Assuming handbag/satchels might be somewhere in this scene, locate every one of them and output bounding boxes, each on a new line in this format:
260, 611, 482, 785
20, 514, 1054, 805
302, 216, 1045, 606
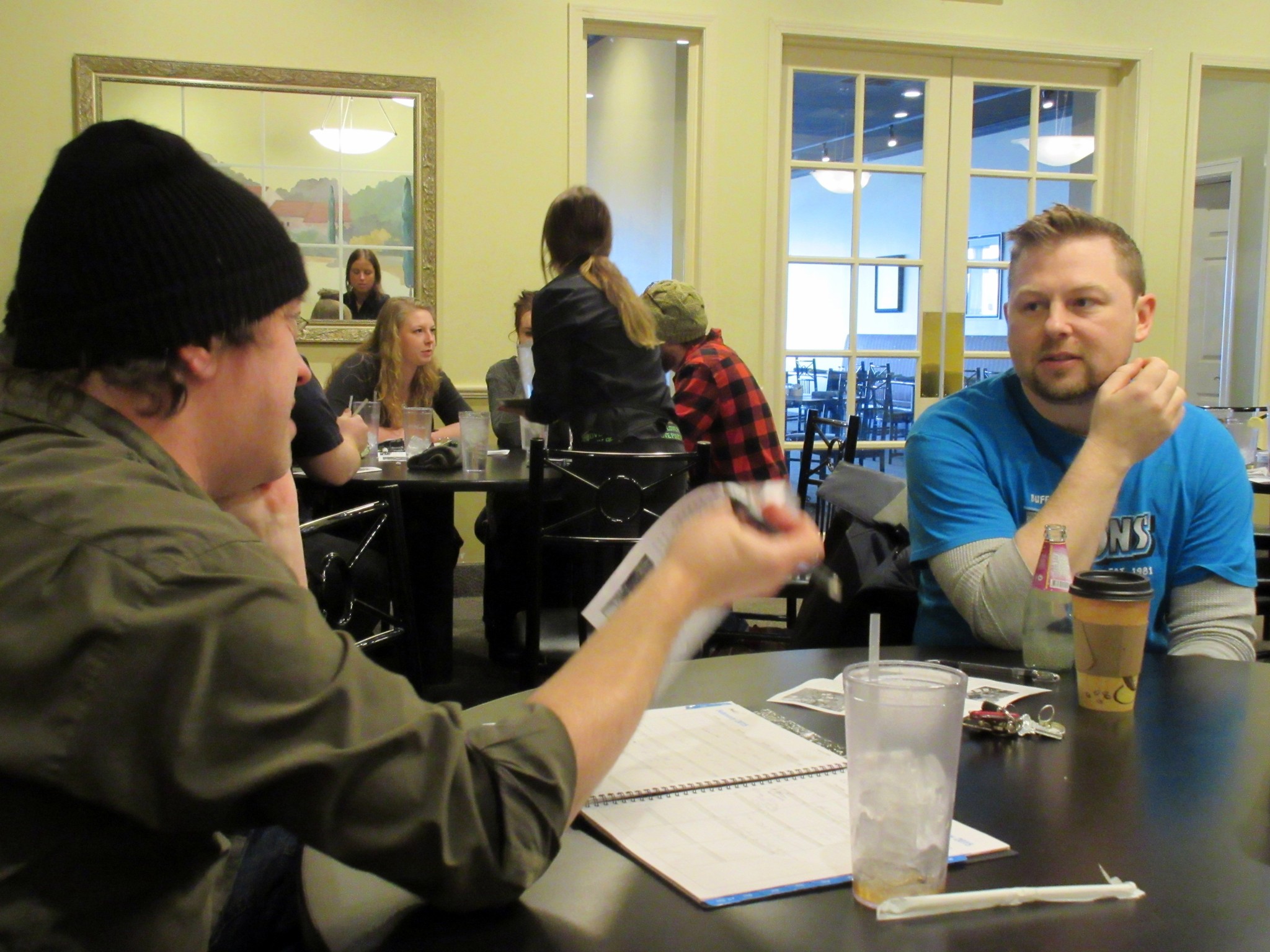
782, 508, 921, 649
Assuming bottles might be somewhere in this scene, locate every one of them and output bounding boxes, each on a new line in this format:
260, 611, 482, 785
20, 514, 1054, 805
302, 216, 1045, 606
1020, 524, 1075, 671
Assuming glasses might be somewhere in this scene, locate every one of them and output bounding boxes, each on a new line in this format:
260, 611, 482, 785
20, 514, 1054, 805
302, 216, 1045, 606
643, 281, 666, 317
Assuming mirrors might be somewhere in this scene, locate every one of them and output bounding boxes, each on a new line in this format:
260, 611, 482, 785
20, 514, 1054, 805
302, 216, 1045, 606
73, 54, 436, 343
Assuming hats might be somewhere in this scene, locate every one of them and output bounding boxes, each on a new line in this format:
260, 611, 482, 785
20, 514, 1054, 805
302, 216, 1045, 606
2, 118, 310, 374
640, 279, 708, 344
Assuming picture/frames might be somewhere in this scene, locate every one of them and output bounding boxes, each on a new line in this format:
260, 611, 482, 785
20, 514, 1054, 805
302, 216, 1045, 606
875, 254, 903, 313
966, 233, 1003, 318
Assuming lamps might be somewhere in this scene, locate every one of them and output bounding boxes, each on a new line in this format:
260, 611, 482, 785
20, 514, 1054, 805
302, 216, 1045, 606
1011, 90, 1095, 166
809, 124, 871, 193
308, 96, 397, 154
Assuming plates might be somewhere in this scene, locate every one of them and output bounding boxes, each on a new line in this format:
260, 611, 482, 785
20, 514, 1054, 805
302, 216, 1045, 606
1249, 478, 1270, 484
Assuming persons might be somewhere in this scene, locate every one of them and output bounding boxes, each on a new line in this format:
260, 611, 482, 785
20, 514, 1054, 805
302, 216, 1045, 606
906, 204, 1259, 660
0, 119, 828, 952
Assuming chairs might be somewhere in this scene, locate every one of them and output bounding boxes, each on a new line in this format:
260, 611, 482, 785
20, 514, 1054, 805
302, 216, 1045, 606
521, 356, 999, 680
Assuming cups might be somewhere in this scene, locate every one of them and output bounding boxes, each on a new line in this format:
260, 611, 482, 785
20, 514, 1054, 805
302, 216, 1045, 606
459, 411, 490, 473
843, 660, 969, 910
1226, 419, 1259, 469
351, 401, 381, 459
400, 407, 434, 461
1069, 569, 1156, 714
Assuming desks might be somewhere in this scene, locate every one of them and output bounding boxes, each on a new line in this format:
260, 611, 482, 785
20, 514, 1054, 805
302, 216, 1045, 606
289, 436, 556, 487
786, 394, 865, 432
300, 646, 1270, 952
